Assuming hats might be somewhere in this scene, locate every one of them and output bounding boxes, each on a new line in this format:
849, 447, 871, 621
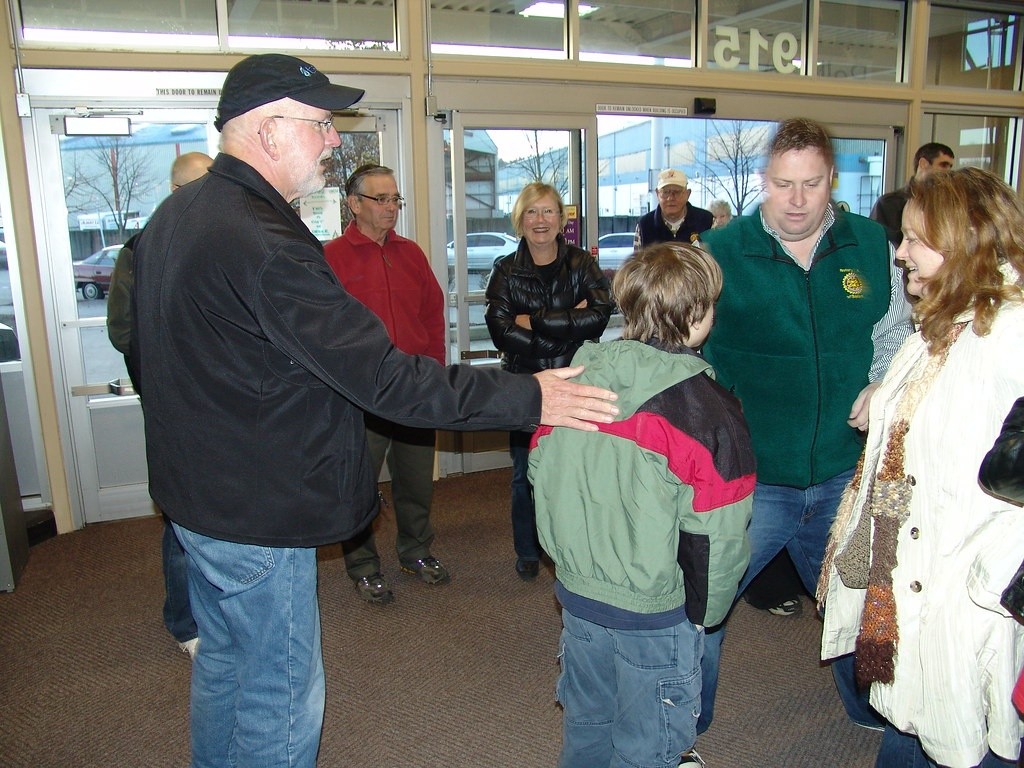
213, 53, 366, 133
656, 169, 688, 191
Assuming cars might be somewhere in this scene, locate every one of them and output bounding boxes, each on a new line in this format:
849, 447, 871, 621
582, 233, 638, 274
0, 241, 8, 269
0, 323, 21, 362
447, 230, 524, 276
73, 243, 126, 300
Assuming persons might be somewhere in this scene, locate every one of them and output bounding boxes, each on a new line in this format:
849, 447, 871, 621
633, 169, 713, 253
677, 119, 914, 768
709, 200, 733, 230
744, 141, 1024, 767
129, 53, 620, 768
526, 240, 755, 768
318, 165, 450, 609
107, 151, 215, 657
485, 183, 610, 582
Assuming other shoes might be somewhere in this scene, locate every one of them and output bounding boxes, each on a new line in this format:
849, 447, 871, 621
178, 636, 199, 658
676, 749, 705, 768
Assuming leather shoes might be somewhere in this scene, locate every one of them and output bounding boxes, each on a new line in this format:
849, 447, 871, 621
517, 558, 540, 579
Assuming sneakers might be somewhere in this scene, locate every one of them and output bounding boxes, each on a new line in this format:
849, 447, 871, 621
770, 598, 803, 615
357, 572, 394, 605
400, 553, 449, 584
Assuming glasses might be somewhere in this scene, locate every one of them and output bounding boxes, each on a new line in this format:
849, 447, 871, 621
929, 160, 954, 170
659, 187, 687, 198
356, 192, 406, 204
258, 115, 334, 133
